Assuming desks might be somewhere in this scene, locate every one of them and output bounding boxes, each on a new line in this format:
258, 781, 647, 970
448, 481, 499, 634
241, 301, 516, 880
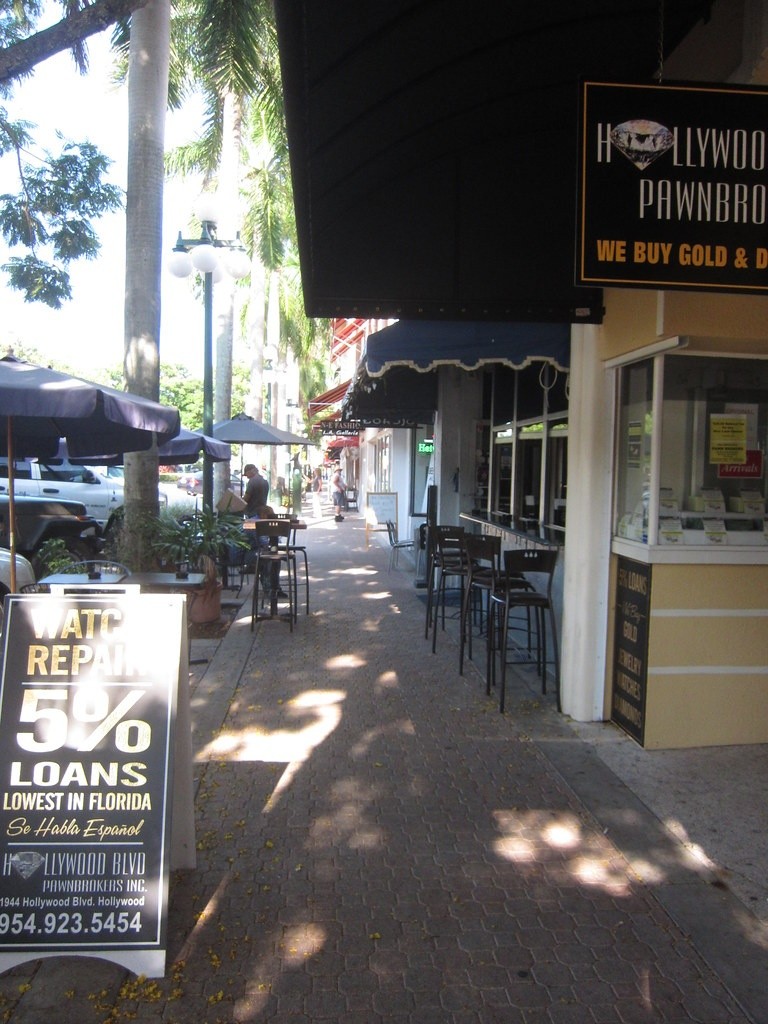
242, 518, 307, 534
38, 572, 209, 664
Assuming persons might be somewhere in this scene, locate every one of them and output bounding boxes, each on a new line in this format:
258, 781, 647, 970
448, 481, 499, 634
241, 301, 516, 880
226, 506, 288, 599
305, 467, 323, 517
241, 464, 269, 519
331, 467, 346, 522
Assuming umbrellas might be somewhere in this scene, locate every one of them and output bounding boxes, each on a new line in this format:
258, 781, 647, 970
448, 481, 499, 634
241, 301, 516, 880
13, 426, 231, 465
0, 348, 181, 594
193, 410, 316, 497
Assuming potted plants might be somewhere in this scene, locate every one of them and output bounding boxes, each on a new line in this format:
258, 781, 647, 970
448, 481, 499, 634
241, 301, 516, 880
150, 494, 252, 624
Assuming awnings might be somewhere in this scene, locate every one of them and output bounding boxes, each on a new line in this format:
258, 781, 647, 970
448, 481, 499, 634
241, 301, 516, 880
327, 436, 359, 450
340, 318, 569, 424
329, 317, 368, 364
311, 410, 342, 434
307, 377, 352, 417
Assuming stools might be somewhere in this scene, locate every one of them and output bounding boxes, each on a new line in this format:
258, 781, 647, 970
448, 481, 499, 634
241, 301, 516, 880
261, 513, 310, 615
429, 531, 490, 656
458, 538, 541, 687
485, 549, 563, 715
250, 521, 298, 633
463, 532, 533, 661
424, 525, 483, 640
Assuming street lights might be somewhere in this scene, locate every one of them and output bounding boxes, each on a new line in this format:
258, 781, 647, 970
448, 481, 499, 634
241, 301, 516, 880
167, 221, 252, 525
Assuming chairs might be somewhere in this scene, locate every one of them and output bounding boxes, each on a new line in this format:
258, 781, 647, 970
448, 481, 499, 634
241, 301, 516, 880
385, 520, 418, 576
19, 583, 104, 594
388, 519, 417, 568
343, 489, 359, 513
60, 560, 131, 575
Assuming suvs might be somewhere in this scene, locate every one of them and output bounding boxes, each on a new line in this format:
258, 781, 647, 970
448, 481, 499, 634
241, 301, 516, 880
0, 495, 105, 577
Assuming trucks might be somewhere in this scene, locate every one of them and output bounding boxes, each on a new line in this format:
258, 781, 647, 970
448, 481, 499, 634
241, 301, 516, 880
0, 455, 168, 542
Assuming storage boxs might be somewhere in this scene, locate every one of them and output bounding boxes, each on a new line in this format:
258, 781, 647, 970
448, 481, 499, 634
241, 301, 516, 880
216, 487, 248, 513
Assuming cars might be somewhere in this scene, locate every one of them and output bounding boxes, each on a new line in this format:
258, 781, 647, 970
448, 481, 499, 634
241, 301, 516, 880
176, 470, 245, 496
0, 546, 37, 640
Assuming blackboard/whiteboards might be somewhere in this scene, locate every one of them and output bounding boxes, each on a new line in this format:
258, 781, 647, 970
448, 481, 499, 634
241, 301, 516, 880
366, 492, 398, 532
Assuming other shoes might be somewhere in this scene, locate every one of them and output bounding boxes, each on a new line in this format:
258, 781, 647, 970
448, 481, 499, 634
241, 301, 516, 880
277, 591, 288, 598
312, 515, 322, 519
335, 514, 344, 522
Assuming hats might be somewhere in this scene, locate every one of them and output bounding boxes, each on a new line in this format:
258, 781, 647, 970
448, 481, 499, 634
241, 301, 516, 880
242, 464, 258, 476
333, 465, 342, 473
